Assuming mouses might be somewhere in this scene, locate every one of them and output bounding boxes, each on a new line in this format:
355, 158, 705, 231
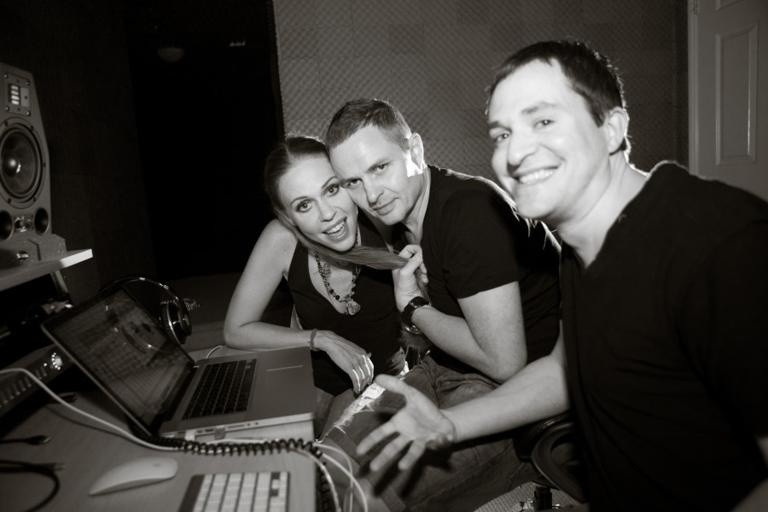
92, 454, 178, 493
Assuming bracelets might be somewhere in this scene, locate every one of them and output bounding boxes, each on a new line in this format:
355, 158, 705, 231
399, 296, 432, 335
308, 329, 320, 352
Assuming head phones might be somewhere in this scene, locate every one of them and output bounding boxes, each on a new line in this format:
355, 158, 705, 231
104, 273, 191, 345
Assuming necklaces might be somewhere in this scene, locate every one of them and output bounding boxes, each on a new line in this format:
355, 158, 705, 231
310, 249, 363, 317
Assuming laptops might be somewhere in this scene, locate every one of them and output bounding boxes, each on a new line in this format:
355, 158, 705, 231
41, 282, 318, 437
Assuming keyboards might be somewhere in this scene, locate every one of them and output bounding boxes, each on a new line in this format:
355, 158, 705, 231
176, 470, 289, 512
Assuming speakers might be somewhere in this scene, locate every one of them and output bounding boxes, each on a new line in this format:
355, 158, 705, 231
1, 65, 68, 261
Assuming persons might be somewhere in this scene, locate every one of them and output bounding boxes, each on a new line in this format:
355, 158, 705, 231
353, 36, 766, 510
221, 135, 433, 435
310, 96, 570, 510
